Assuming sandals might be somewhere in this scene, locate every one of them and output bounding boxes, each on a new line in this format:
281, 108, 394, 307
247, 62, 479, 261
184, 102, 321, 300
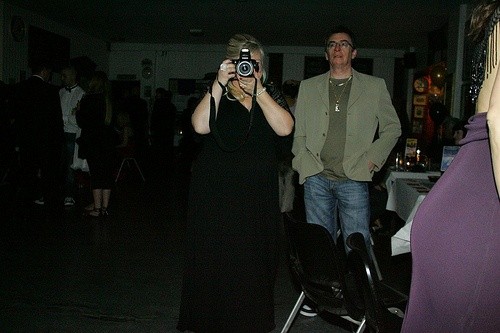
82, 208, 110, 219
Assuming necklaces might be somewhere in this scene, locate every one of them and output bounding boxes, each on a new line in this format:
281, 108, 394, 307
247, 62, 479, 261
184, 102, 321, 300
330, 75, 352, 112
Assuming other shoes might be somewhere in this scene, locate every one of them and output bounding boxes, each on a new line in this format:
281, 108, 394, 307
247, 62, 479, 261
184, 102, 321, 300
299, 304, 317, 317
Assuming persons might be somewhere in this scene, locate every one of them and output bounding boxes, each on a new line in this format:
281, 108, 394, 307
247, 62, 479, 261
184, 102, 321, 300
176, 34, 295, 333
0, 41, 118, 218
399, 0, 500, 333
291, 27, 402, 316
282, 79, 467, 171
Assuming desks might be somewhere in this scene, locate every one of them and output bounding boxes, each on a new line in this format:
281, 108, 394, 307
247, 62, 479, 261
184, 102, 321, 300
386, 172, 443, 265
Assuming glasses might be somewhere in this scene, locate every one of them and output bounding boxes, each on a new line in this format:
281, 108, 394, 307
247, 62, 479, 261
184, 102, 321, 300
326, 40, 355, 50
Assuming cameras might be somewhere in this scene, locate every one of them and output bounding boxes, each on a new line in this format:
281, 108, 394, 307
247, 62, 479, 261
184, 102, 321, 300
229, 49, 259, 81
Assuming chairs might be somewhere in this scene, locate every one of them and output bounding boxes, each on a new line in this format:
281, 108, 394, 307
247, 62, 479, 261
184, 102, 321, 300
281, 212, 404, 333
344, 231, 403, 333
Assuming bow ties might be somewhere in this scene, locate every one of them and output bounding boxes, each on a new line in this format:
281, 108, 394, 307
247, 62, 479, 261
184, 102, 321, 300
64, 87, 72, 92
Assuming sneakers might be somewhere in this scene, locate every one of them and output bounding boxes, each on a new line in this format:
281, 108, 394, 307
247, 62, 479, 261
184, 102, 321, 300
35, 198, 45, 206
64, 196, 75, 207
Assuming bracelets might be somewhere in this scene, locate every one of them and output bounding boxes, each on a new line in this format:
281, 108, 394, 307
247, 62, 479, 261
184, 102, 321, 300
257, 87, 266, 96
